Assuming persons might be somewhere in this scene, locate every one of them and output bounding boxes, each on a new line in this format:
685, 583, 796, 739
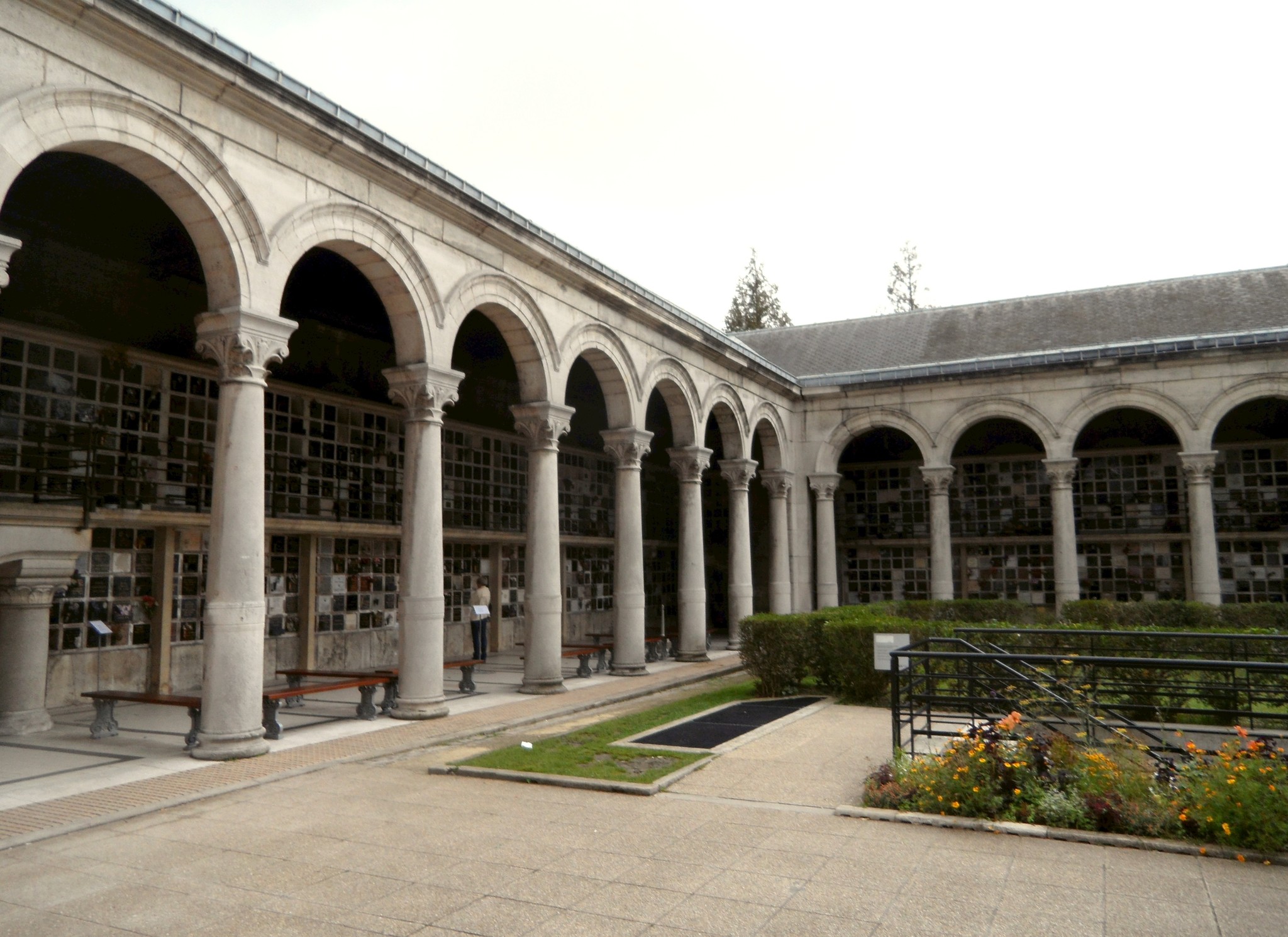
470, 578, 491, 663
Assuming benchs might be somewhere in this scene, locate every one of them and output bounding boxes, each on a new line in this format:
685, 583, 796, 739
261, 676, 389, 740
514, 627, 716, 679
275, 668, 397, 717
80, 690, 202, 751
374, 658, 484, 708
